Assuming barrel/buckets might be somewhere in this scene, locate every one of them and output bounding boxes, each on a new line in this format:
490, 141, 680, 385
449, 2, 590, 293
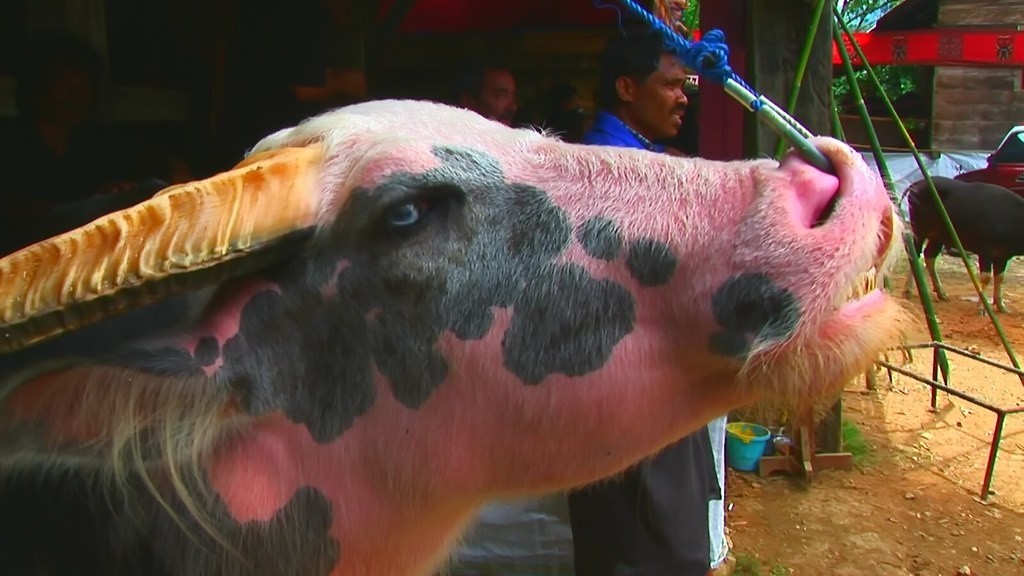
725, 422, 771, 470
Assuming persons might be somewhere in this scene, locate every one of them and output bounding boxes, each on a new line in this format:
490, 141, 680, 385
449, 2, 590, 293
569, 25, 721, 576
0, 34, 109, 257
444, 50, 524, 126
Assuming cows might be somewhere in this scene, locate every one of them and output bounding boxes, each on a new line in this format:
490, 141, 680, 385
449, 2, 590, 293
0, 98, 1023, 576
